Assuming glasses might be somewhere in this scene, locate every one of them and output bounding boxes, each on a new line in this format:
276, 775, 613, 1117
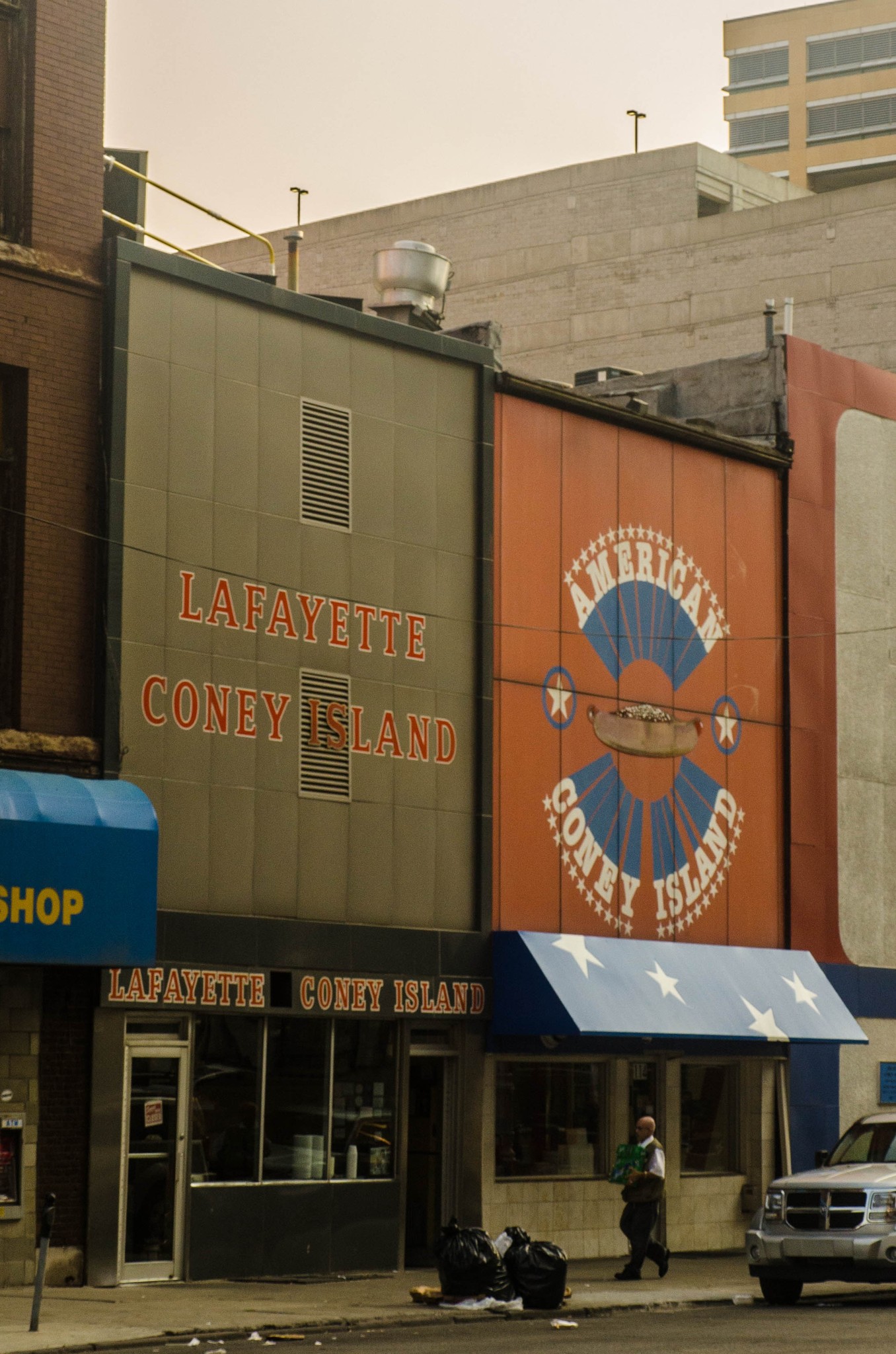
635, 1126, 645, 1130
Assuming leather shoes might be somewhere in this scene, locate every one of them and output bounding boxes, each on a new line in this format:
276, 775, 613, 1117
615, 1266, 641, 1281
659, 1249, 670, 1278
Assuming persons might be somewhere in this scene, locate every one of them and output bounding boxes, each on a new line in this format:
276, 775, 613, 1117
613, 1115, 672, 1281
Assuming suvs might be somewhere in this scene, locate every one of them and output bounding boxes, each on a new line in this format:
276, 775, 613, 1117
746, 1108, 896, 1302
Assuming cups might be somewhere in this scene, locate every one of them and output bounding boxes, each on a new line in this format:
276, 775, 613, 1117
331, 1157, 334, 1178
295, 1133, 324, 1180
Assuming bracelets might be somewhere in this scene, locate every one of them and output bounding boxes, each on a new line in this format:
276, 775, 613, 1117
643, 1171, 647, 1179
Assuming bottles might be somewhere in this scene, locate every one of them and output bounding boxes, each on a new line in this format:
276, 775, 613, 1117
347, 1146, 358, 1179
